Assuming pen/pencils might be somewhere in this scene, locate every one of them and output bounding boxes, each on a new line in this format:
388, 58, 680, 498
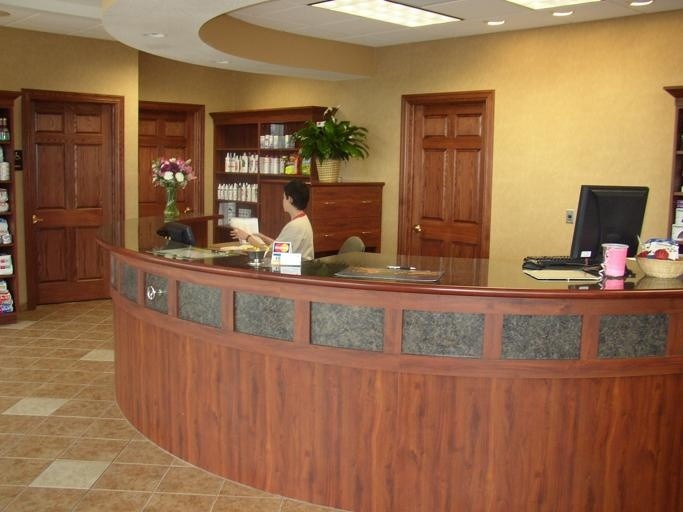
387, 265, 416, 270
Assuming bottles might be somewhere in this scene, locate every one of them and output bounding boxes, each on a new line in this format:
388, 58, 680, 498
0, 118, 10, 144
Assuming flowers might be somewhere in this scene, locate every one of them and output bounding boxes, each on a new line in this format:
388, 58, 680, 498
152, 158, 197, 188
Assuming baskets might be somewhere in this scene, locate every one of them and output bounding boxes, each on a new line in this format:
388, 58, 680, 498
634, 254, 683, 278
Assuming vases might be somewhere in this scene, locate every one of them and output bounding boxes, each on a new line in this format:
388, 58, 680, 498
164, 184, 179, 219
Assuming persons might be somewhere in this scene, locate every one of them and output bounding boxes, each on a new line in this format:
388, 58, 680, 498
230, 180, 315, 261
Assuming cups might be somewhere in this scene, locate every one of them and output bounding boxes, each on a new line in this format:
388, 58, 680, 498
604, 279, 624, 290
602, 243, 629, 277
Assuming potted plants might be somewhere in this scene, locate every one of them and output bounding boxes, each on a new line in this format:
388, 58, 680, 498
295, 105, 371, 180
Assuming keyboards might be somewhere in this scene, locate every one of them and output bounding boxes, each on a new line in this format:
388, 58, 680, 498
523, 255, 584, 266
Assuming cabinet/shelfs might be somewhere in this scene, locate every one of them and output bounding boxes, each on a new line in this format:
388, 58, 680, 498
663, 84, 683, 255
259, 180, 384, 258
209, 105, 336, 250
1, 90, 24, 325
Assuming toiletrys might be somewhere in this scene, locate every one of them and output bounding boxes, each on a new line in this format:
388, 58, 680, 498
217, 182, 258, 203
225, 152, 259, 173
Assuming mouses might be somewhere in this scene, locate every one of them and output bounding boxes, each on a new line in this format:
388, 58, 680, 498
522, 262, 541, 270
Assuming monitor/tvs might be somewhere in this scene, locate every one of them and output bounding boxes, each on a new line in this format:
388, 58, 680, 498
166, 221, 195, 245
571, 184, 650, 259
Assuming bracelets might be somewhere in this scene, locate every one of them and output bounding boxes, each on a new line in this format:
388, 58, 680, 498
246, 235, 251, 242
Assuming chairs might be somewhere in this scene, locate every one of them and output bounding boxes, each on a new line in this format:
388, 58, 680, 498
337, 236, 366, 254
